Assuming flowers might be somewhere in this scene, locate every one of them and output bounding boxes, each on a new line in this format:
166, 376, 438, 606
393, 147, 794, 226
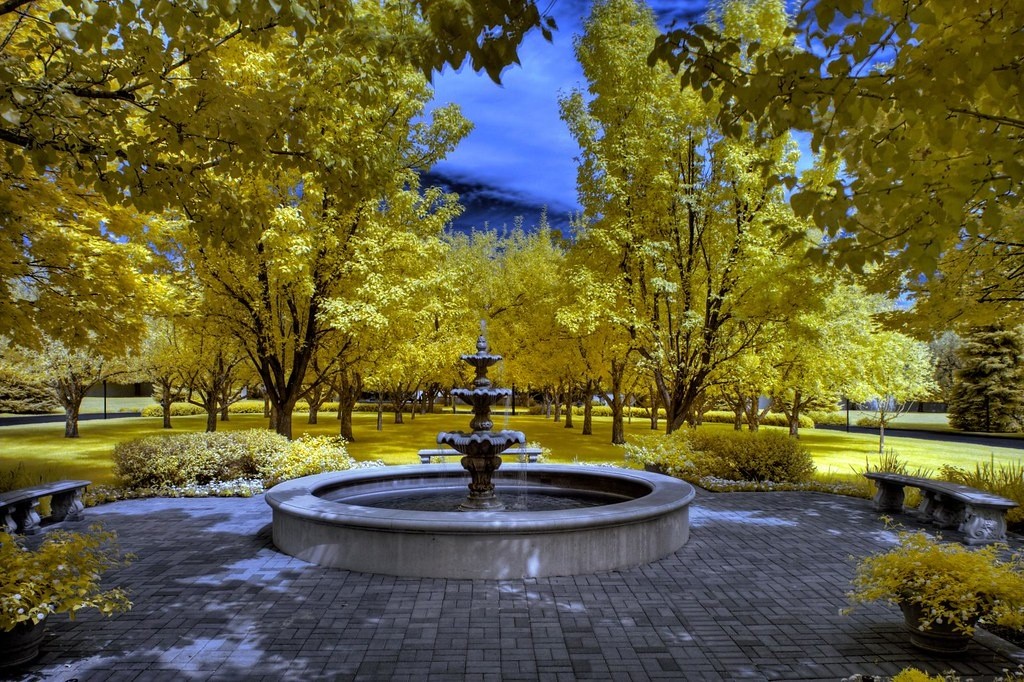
0, 521, 138, 632
838, 515, 1024, 633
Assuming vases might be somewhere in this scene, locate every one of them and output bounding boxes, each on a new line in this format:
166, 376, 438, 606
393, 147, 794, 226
0, 612, 50, 668
895, 585, 994, 653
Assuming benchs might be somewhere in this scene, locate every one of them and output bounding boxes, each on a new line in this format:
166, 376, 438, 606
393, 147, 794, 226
417, 448, 542, 465
0, 480, 92, 536
864, 472, 1019, 546
